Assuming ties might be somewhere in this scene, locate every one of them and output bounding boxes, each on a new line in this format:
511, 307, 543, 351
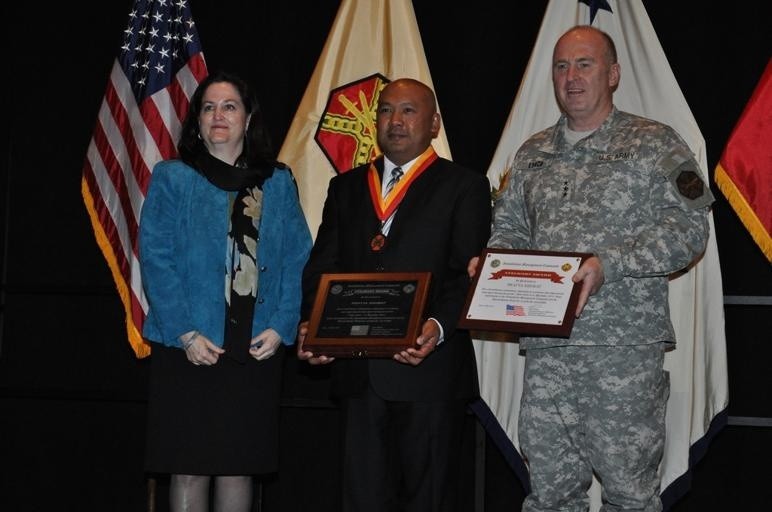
381, 168, 404, 236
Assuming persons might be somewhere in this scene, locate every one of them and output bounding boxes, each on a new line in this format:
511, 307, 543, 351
467, 26, 717, 512
134, 73, 312, 512
296, 77, 493, 512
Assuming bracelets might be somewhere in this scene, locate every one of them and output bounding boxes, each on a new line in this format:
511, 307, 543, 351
185, 331, 200, 350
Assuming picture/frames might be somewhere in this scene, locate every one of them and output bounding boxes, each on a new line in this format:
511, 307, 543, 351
303, 271, 431, 359
457, 247, 592, 339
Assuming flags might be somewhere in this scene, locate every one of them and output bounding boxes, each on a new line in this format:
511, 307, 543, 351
78, 1, 213, 360
713, 59, 772, 263
275, 0, 456, 244
475, 1, 739, 511
